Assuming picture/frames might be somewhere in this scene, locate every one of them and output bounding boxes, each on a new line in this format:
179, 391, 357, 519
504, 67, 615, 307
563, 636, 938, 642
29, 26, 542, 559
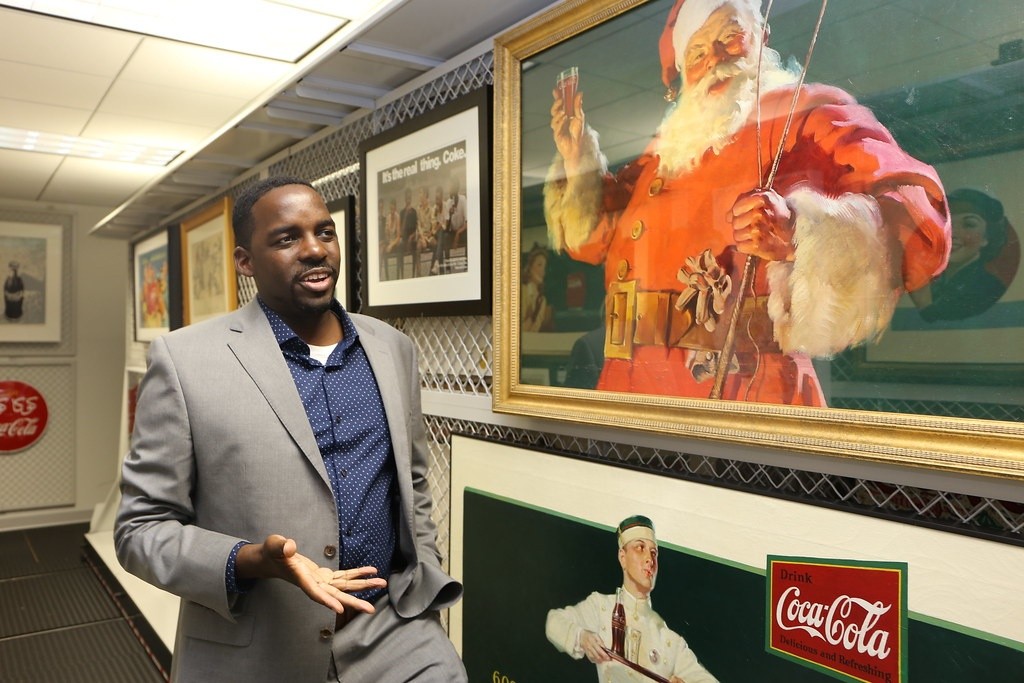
0, 221, 63, 343
519, 199, 605, 357
446, 432, 1023, 683
490, 1, 1024, 483
324, 194, 358, 313
132, 223, 184, 342
359, 83, 492, 319
180, 197, 239, 328
828, 140, 1023, 388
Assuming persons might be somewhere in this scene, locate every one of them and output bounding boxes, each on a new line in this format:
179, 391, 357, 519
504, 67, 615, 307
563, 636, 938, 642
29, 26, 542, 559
564, 302, 605, 390
377, 178, 466, 281
4, 262, 25, 323
113, 178, 467, 683
140, 261, 168, 328
